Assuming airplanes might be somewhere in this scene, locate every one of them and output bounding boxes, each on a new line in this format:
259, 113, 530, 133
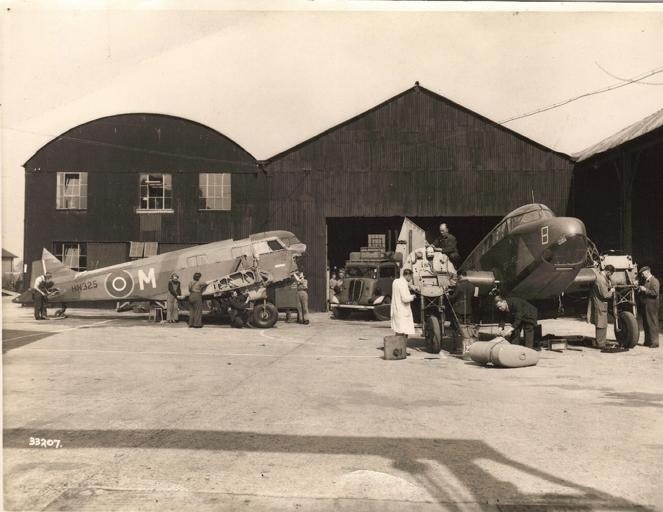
423, 203, 639, 351
12, 229, 307, 328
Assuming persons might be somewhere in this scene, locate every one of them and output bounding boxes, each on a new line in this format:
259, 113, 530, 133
637, 265, 660, 347
446, 269, 475, 331
391, 267, 417, 357
229, 291, 250, 327
492, 294, 539, 353
591, 264, 616, 346
426, 223, 462, 268
330, 270, 345, 305
167, 272, 207, 328
292, 271, 311, 324
33, 271, 52, 320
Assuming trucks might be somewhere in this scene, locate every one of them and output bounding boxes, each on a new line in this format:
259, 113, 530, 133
330, 251, 403, 321
